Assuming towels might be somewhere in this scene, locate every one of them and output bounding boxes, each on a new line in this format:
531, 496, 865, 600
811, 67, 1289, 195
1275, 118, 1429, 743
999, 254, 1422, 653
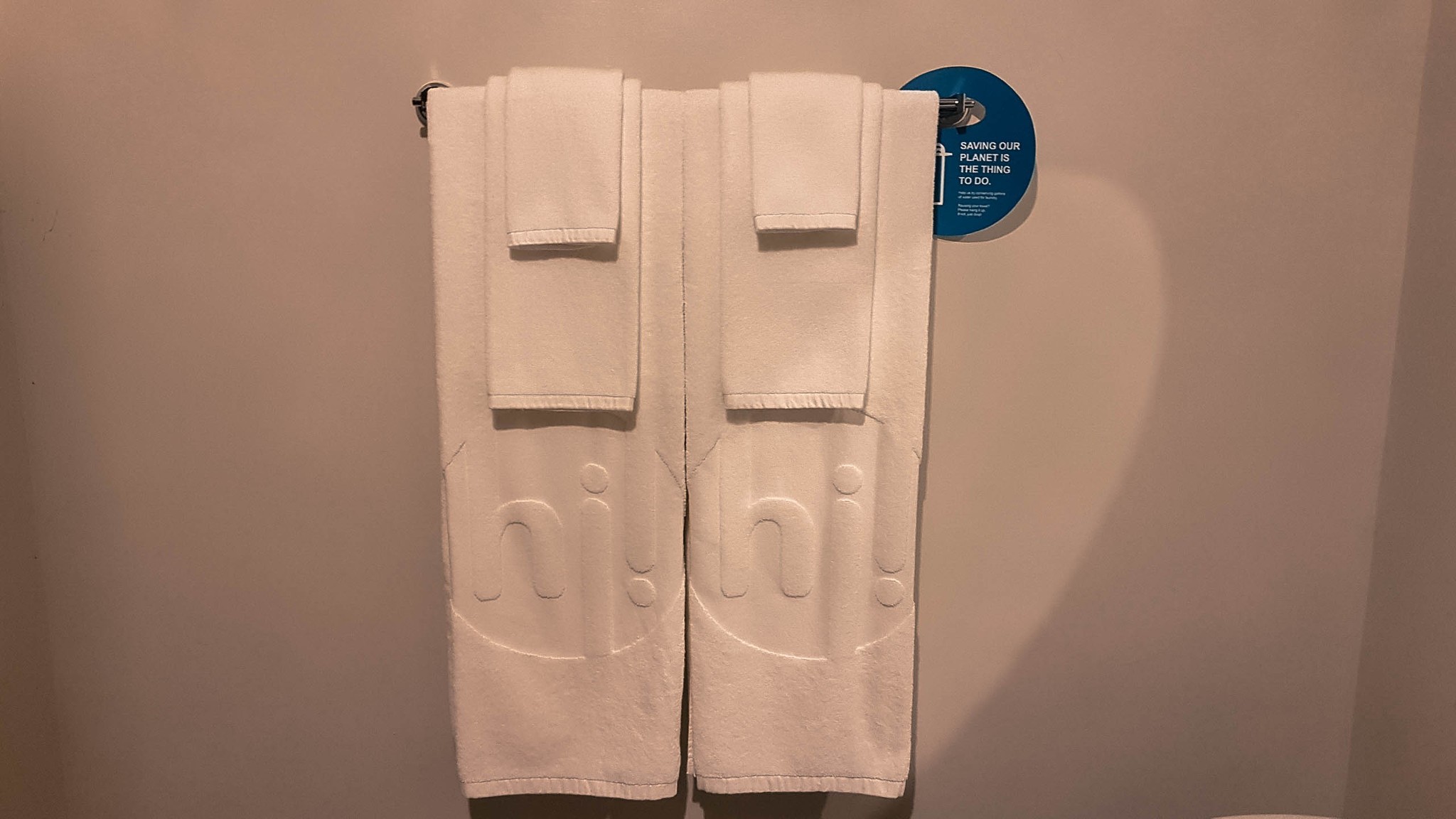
717, 80, 886, 414
486, 72, 644, 414
680, 86, 941, 806
422, 75, 690, 805
746, 70, 866, 235
503, 64, 626, 252
